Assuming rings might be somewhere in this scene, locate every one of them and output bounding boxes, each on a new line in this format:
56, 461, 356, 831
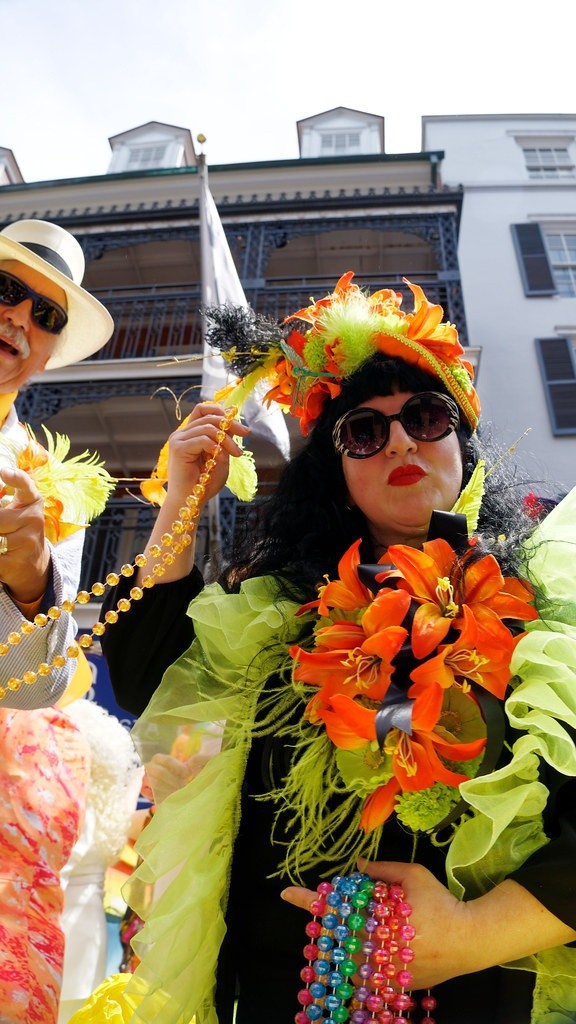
0, 537, 7, 554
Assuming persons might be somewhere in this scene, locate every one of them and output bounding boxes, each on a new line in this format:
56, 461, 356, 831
95, 273, 575, 1024
130, 719, 226, 957
0, 221, 138, 1024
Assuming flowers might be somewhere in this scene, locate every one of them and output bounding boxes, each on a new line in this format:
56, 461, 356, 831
0, 419, 115, 543
290, 542, 539, 835
260, 275, 471, 426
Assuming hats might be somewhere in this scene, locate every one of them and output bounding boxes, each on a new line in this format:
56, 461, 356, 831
0, 218, 115, 370
134, 270, 485, 508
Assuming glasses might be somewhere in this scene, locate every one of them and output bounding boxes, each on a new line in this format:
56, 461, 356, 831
0, 270, 69, 336
327, 390, 462, 460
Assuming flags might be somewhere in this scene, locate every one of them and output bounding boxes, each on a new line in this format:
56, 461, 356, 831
200, 174, 290, 461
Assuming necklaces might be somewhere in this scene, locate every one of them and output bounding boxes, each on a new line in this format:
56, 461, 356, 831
293, 871, 436, 1024
0, 406, 236, 700
